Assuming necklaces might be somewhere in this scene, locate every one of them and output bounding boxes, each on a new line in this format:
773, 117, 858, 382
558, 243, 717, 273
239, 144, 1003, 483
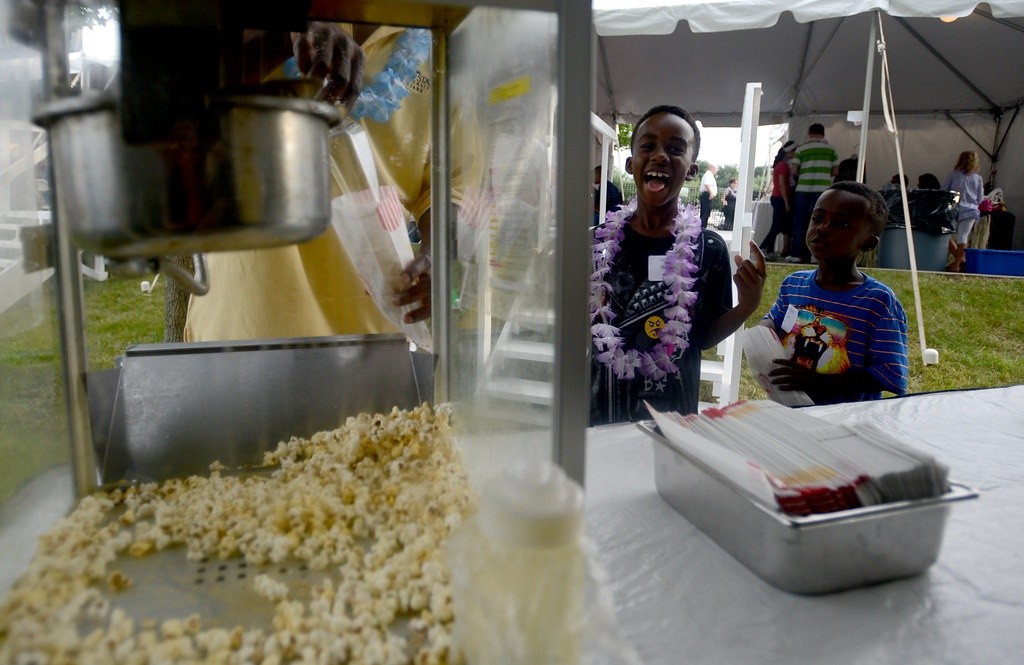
591, 196, 702, 379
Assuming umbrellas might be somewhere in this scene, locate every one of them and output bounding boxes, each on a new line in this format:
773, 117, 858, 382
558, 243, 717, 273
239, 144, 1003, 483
0, 51, 109, 123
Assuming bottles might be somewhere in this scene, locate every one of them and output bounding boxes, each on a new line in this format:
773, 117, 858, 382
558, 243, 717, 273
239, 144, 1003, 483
451, 460, 590, 665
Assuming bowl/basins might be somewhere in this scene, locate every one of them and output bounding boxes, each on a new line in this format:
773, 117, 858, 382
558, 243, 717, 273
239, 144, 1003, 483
33, 80, 343, 254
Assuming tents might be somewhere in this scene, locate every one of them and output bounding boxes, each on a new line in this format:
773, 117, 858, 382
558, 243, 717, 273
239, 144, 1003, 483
553, 1, 1024, 254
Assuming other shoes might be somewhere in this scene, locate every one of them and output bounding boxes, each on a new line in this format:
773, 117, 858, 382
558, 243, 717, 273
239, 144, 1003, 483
944, 262, 959, 273
784, 255, 800, 262
796, 259, 811, 264
759, 252, 778, 262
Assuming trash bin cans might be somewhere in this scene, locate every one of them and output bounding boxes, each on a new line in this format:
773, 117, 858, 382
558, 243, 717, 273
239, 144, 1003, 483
877, 188, 958, 270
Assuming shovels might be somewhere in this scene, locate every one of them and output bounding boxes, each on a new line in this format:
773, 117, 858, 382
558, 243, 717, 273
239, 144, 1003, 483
323, 85, 379, 209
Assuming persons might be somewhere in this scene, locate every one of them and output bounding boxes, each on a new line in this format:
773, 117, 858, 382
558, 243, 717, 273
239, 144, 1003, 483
891, 175, 909, 189
592, 188, 599, 225
591, 106, 767, 427
593, 166, 630, 212
917, 173, 941, 190
758, 140, 799, 260
757, 181, 908, 409
721, 178, 738, 230
789, 123, 840, 262
941, 151, 984, 272
183, 19, 483, 413
700, 164, 718, 230
